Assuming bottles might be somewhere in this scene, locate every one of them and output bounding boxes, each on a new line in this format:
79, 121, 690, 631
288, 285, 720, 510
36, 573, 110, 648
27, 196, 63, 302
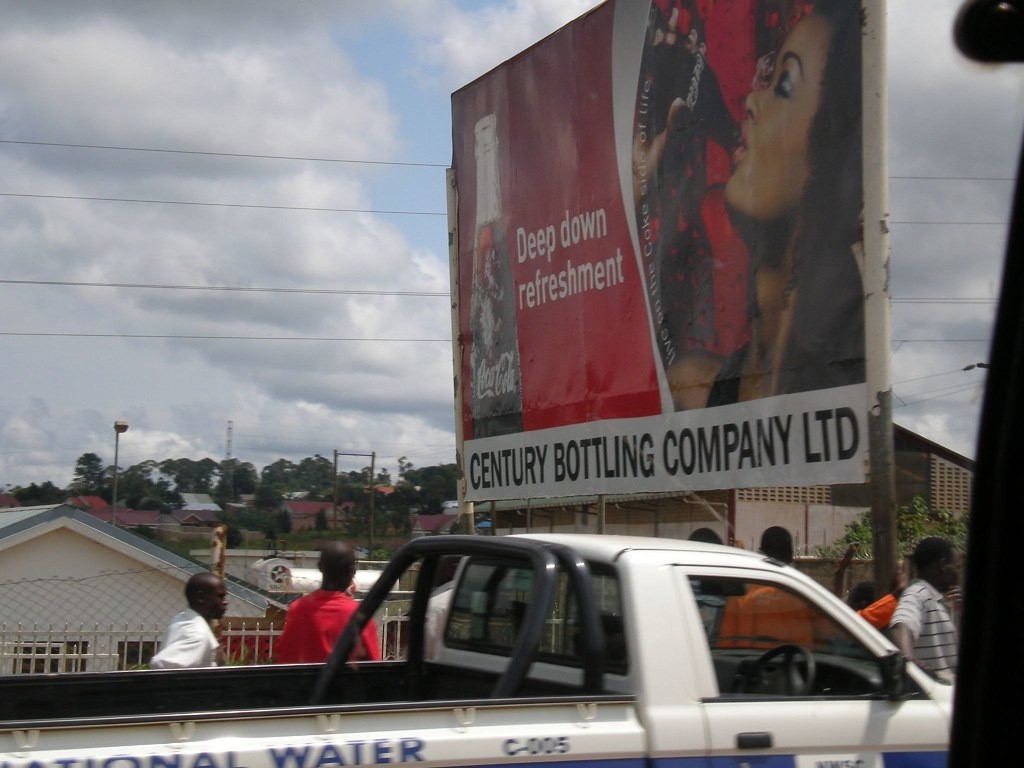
469, 113, 522, 421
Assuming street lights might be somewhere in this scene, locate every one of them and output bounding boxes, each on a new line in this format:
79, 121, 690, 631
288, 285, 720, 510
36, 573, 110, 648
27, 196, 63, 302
110, 419, 130, 526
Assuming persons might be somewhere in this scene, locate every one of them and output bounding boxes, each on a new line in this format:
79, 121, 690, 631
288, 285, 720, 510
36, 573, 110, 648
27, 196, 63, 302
148, 572, 227, 669
272, 542, 380, 663
424, 554, 461, 660
687, 528, 747, 595
889, 538, 958, 683
722, 526, 906, 654
631, 0, 863, 409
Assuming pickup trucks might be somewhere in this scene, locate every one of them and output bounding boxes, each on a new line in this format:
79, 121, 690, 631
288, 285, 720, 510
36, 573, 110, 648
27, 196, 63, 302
0, 526, 960, 767
